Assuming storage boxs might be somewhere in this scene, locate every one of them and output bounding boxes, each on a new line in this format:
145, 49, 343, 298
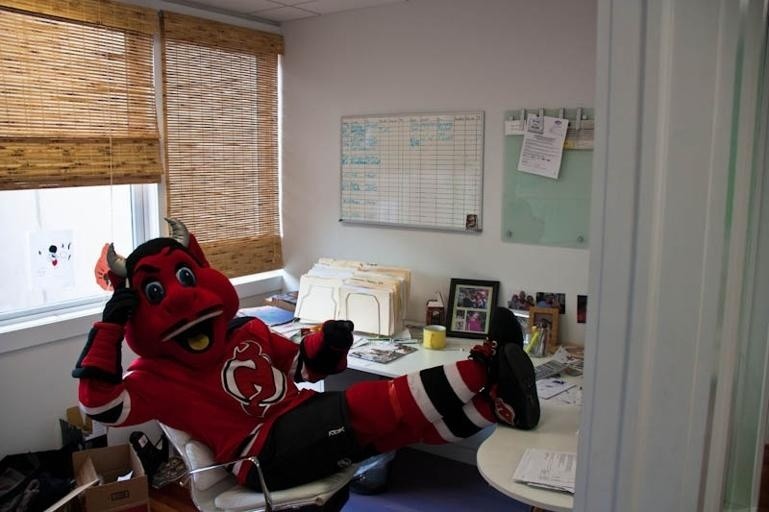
57, 402, 110, 456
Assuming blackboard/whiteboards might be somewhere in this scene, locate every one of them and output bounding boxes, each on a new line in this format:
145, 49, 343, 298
338, 112, 486, 235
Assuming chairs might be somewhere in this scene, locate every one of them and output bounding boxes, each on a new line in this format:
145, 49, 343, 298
149, 413, 365, 512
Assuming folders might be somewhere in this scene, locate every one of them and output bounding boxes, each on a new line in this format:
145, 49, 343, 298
236, 305, 296, 327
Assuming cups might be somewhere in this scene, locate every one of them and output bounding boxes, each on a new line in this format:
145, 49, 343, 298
516, 319, 551, 357
421, 324, 448, 351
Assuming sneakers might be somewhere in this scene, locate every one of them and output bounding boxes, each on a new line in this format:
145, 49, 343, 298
481, 341, 541, 433
467, 306, 526, 375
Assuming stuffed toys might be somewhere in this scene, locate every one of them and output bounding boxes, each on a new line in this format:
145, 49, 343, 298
70, 214, 539, 493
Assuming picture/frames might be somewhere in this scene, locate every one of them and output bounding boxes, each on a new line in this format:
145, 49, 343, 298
510, 307, 530, 345
527, 305, 559, 356
444, 276, 500, 340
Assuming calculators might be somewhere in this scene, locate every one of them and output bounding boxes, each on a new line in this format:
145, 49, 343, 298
534, 360, 568, 381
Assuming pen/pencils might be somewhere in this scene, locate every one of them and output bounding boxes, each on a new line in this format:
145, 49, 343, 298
525, 321, 550, 357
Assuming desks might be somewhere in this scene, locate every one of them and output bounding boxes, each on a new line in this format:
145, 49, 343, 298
268, 309, 584, 511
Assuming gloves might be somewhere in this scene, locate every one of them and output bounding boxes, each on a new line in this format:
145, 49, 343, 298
322, 318, 354, 352
100, 284, 141, 324
